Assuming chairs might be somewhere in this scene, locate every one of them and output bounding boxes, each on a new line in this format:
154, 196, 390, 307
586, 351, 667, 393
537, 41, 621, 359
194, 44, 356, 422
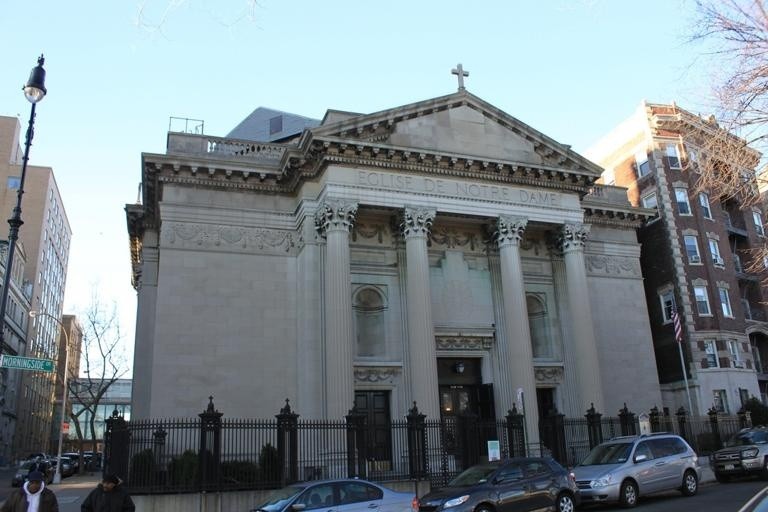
311, 493, 321, 505
325, 495, 333, 506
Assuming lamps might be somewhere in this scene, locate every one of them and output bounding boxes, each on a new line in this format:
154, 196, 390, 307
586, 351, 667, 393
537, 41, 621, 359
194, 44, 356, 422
454, 361, 466, 375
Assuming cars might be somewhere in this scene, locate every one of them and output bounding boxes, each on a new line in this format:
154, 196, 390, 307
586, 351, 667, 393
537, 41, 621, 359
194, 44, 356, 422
737, 486, 768, 512
12, 451, 102, 488
250, 479, 420, 512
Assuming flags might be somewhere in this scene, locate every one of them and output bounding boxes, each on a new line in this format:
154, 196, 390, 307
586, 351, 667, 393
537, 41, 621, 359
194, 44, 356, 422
671, 296, 682, 342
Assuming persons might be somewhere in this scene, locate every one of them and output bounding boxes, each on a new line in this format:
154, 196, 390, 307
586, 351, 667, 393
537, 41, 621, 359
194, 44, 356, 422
305, 493, 321, 510
80, 472, 136, 511
0, 471, 59, 511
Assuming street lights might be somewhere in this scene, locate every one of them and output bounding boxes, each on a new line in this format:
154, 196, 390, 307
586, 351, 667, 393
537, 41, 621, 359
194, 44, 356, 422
0, 52, 47, 358
29, 311, 69, 484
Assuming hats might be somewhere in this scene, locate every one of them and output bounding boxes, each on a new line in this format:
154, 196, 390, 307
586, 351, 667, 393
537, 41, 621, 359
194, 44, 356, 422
28, 471, 43, 481
103, 475, 119, 483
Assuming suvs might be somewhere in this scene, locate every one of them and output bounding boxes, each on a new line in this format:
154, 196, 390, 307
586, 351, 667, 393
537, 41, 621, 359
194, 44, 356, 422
570, 432, 702, 507
709, 425, 768, 484
418, 456, 582, 512
